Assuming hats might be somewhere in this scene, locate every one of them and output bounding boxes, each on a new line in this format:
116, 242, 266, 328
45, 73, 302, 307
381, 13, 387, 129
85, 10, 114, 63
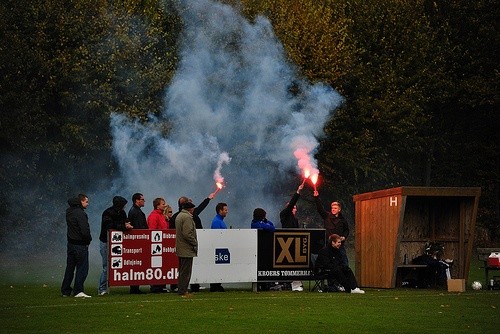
182, 202, 195, 208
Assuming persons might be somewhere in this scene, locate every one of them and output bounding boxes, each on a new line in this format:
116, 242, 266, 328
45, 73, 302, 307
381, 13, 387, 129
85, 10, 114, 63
280, 184, 300, 291
128, 193, 149, 293
147, 193, 214, 293
251, 208, 275, 292
210, 203, 232, 292
61, 194, 92, 298
98, 196, 133, 296
314, 191, 349, 292
314, 234, 365, 293
175, 202, 198, 298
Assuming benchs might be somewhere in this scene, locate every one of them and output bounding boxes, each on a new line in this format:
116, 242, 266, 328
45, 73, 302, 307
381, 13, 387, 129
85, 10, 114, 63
476, 248, 500, 288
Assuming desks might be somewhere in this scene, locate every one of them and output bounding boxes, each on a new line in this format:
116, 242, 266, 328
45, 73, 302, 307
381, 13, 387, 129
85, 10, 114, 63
397, 265, 426, 289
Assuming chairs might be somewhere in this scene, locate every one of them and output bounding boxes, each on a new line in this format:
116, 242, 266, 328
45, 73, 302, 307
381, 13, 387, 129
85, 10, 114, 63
309, 253, 336, 292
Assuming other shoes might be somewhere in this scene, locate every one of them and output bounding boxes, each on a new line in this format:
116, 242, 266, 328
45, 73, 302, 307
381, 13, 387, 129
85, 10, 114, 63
351, 288, 365, 293
180, 292, 192, 298
74, 292, 92, 298
97, 291, 108, 295
